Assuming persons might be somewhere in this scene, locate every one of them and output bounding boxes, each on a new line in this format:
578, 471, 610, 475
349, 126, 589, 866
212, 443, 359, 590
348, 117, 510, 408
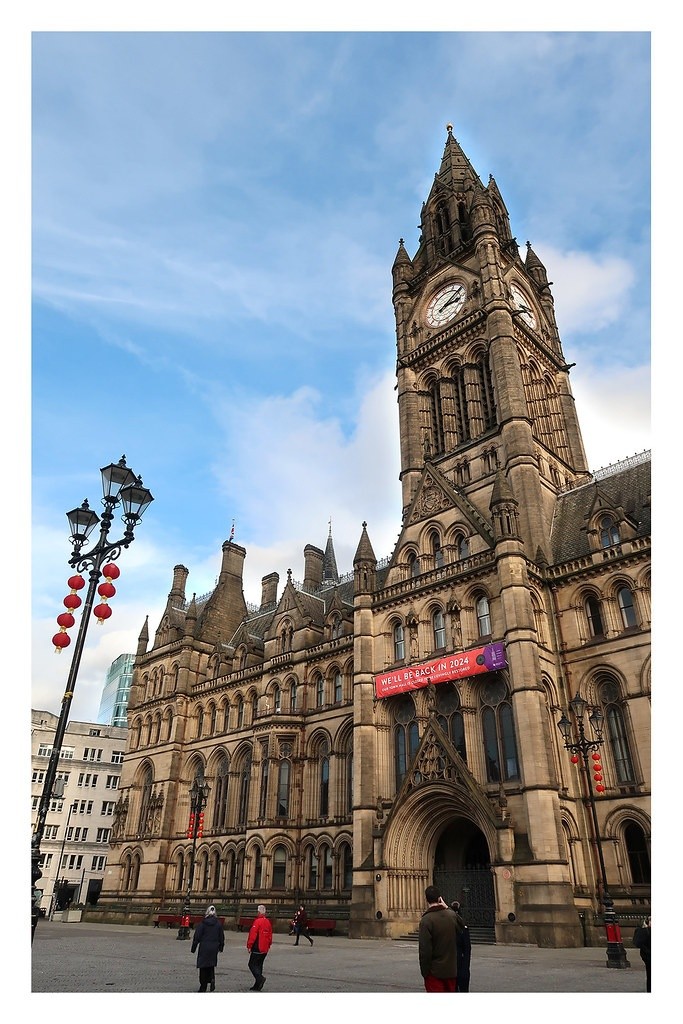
190, 905, 225, 993
419, 886, 471, 993
247, 905, 272, 992
633, 914, 651, 993
289, 904, 313, 947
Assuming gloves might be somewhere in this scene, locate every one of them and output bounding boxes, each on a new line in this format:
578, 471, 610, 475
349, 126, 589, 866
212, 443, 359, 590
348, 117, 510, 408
219, 946, 224, 952
190, 947, 196, 953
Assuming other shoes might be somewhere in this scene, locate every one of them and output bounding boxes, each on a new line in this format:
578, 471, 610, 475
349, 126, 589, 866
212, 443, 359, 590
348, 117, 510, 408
293, 943, 299, 946
249, 980, 259, 990
257, 976, 266, 991
310, 939, 314, 947
197, 986, 208, 992
209, 979, 215, 992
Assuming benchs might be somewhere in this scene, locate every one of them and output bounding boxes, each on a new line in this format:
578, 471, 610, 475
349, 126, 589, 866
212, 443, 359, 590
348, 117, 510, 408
190, 916, 225, 930
152, 914, 181, 929
235, 917, 255, 932
307, 919, 336, 937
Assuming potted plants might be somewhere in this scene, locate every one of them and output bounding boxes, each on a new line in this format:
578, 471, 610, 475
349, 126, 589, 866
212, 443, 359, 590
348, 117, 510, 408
62, 901, 84, 923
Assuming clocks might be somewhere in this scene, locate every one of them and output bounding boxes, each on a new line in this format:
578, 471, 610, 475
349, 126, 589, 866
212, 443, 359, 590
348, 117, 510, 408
417, 275, 470, 329
510, 277, 541, 334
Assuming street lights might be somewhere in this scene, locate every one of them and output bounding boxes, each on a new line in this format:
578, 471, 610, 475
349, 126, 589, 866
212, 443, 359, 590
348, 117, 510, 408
31, 444, 158, 882
176, 765, 214, 941
550, 691, 632, 969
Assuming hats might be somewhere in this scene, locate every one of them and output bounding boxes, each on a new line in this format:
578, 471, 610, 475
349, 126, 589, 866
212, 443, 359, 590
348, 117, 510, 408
205, 905, 216, 915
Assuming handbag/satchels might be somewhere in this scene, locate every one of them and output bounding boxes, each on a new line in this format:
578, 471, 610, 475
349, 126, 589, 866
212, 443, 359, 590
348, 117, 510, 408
296, 920, 302, 926
